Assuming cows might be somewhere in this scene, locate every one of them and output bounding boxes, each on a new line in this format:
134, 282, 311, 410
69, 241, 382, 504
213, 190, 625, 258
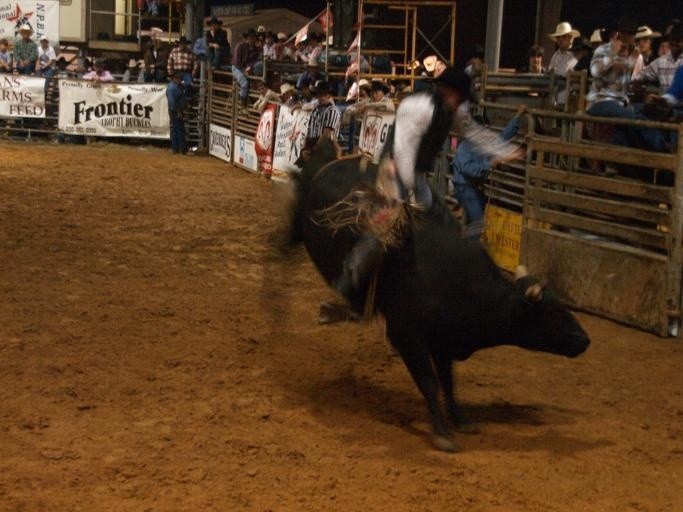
277, 135, 591, 454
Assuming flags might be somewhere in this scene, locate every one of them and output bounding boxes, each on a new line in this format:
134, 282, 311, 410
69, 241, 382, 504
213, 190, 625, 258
320, 10, 333, 31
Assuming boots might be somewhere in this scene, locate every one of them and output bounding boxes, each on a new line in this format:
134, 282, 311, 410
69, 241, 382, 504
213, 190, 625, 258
318, 266, 372, 324
240, 97, 250, 115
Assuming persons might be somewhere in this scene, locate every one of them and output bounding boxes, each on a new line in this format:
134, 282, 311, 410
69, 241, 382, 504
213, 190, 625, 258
193, 15, 409, 169
514, 23, 683, 230
452, 100, 528, 243
0, 18, 198, 157
470, 52, 485, 75
315, 66, 525, 326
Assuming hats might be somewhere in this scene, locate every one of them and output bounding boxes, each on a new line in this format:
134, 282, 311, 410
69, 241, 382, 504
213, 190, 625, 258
420, 66, 479, 104
17, 24, 30, 32
548, 21, 662, 42
359, 78, 411, 97
175, 36, 192, 44
92, 58, 105, 66
423, 55, 437, 73
277, 57, 332, 97
206, 17, 223, 26
41, 35, 49, 41
127, 59, 142, 68
243, 25, 321, 43
168, 71, 183, 77
